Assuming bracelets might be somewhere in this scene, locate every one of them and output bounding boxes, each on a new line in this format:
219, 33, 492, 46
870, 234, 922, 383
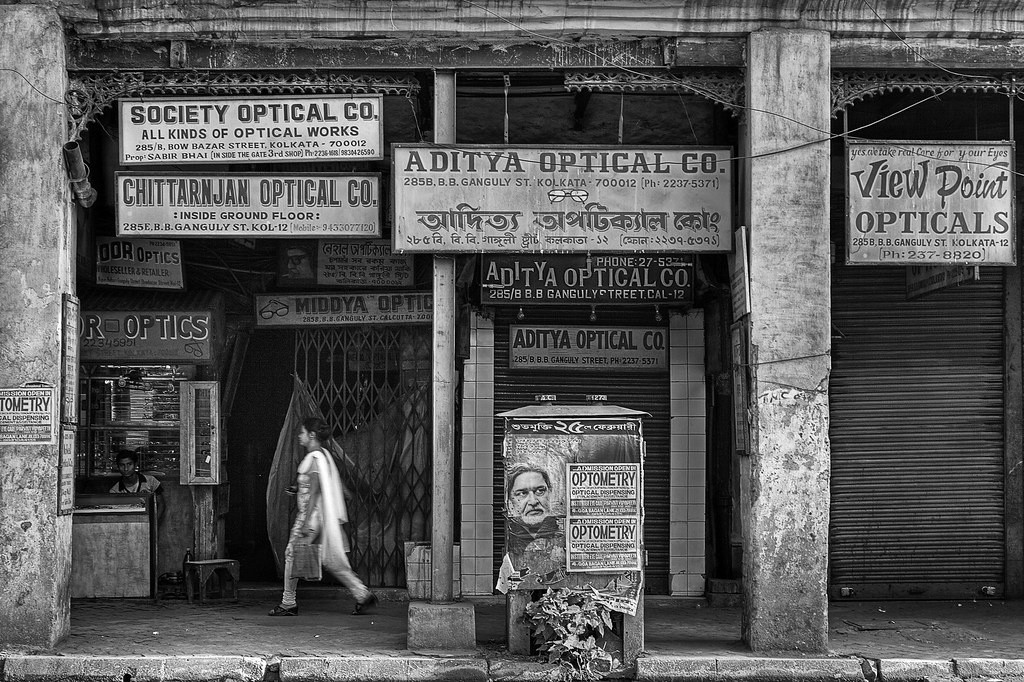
303, 524, 309, 527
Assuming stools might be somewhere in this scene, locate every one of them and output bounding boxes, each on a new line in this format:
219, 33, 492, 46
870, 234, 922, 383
185, 559, 240, 604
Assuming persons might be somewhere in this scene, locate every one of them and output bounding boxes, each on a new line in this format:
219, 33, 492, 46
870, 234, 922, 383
109, 449, 167, 561
268, 417, 377, 616
506, 462, 567, 571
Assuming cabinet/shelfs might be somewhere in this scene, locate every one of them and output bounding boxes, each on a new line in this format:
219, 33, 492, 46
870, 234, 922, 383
92, 377, 188, 475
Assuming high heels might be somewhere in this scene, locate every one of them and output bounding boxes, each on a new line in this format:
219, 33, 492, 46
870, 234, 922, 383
265, 606, 298, 616
352, 594, 378, 615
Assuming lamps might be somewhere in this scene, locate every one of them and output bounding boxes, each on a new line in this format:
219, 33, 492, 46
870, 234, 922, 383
586, 250, 593, 274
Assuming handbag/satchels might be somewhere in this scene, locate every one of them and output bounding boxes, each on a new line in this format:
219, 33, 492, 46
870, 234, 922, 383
290, 535, 323, 581
